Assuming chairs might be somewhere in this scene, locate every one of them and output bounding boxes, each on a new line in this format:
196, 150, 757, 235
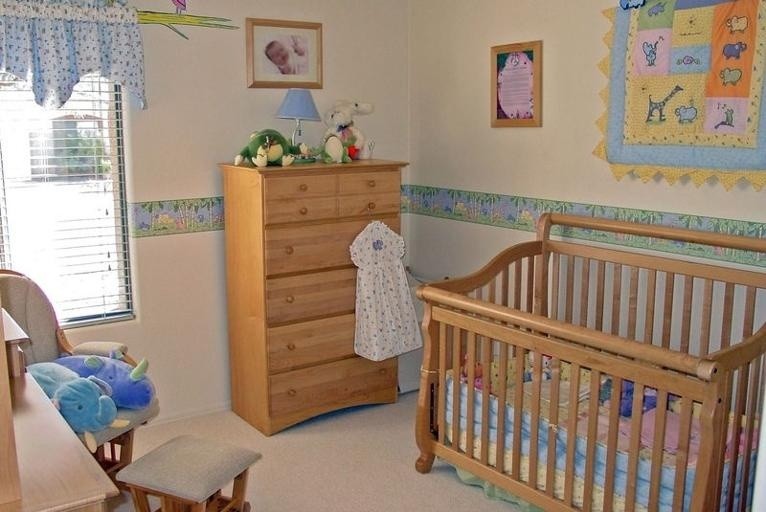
0, 270, 160, 492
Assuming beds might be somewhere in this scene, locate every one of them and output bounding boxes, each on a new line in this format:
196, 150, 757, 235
414, 211, 764, 511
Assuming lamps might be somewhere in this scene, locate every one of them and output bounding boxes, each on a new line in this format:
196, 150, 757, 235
276, 89, 322, 151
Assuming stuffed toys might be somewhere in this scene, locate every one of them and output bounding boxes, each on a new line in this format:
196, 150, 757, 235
463, 348, 551, 390
28, 363, 130, 455
310, 102, 376, 163
234, 128, 308, 168
52, 349, 155, 410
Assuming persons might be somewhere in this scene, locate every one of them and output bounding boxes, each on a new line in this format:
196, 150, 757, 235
265, 39, 309, 77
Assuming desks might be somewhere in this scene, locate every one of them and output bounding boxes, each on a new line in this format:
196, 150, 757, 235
0, 305, 120, 512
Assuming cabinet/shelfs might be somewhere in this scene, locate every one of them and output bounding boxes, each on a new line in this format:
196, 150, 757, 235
219, 159, 409, 439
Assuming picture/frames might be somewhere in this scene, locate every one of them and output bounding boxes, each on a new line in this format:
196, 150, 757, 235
490, 40, 541, 129
245, 17, 324, 89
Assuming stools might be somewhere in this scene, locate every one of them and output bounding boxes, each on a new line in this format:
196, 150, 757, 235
116, 433, 264, 512
